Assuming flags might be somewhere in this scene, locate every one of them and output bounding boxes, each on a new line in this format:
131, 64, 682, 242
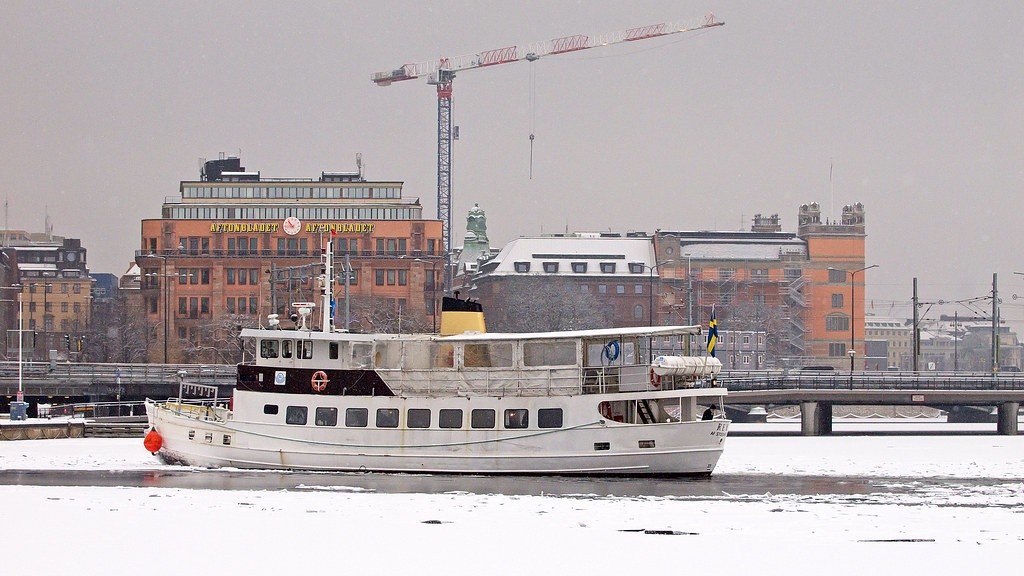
707, 311, 718, 357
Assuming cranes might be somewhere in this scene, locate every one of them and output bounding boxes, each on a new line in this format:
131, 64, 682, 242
368, 9, 725, 255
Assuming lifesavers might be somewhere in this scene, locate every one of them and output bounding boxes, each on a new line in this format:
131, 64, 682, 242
598, 401, 612, 419
311, 371, 328, 391
605, 340, 619, 361
650, 368, 660, 387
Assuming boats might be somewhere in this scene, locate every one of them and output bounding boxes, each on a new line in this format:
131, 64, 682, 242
144, 227, 733, 478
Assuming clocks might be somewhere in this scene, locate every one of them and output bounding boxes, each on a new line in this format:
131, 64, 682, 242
283, 216, 302, 237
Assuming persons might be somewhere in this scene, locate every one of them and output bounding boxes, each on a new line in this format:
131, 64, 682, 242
702, 404, 716, 420
270, 349, 276, 358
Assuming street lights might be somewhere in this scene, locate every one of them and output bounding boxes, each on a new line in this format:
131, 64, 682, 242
825, 263, 879, 389
636, 262, 666, 364
147, 252, 169, 362
